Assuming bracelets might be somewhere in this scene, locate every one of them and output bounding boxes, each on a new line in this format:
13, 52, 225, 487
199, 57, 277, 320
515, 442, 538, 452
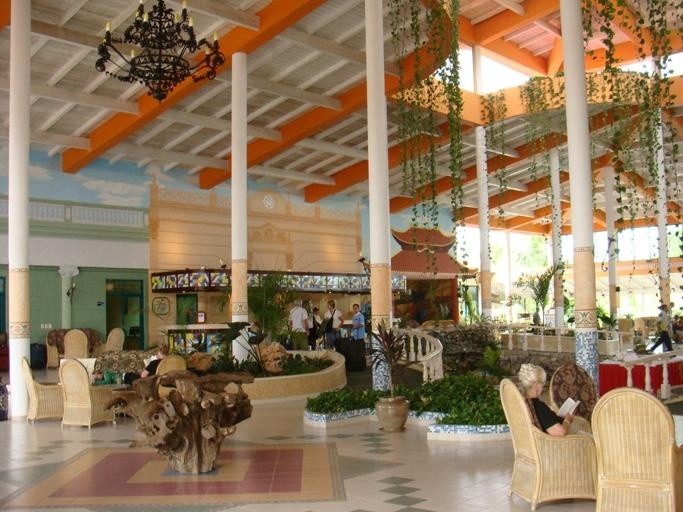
563, 419, 570, 425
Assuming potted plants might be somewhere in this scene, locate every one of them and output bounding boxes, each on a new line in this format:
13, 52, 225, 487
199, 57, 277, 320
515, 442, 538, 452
356, 314, 424, 435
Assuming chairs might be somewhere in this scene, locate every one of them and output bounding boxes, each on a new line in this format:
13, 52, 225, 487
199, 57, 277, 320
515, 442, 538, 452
547, 363, 601, 421
587, 382, 682, 512
493, 371, 599, 512
492, 313, 683, 349
20, 326, 194, 431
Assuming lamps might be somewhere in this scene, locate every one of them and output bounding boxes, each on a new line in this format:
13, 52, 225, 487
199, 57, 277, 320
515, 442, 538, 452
89, 1, 227, 102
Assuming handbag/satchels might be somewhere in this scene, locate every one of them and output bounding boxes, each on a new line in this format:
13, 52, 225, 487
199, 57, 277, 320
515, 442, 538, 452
324, 317, 333, 333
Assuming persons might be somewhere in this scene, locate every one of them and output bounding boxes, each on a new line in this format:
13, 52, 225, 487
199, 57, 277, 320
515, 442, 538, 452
92, 371, 140, 385
140, 346, 169, 378
650, 302, 683, 359
518, 362, 574, 438
286, 299, 366, 372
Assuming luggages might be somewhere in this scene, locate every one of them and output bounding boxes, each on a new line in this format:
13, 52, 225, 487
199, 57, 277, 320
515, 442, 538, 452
337, 327, 366, 372
